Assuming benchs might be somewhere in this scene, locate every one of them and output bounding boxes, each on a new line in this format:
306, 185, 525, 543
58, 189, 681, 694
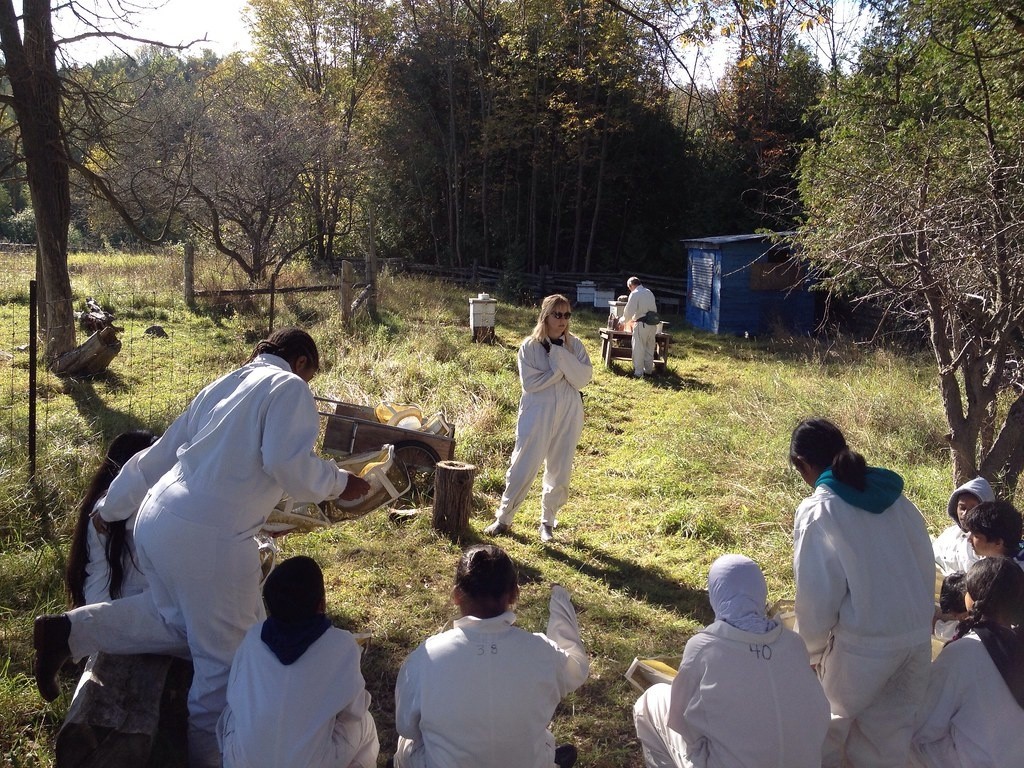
56, 651, 173, 767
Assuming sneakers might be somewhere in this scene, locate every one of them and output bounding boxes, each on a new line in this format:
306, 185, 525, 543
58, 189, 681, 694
484, 521, 508, 537
538, 523, 554, 543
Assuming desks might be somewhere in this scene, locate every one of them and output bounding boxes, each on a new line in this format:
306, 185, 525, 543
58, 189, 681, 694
600, 327, 673, 374
468, 298, 497, 343
576, 283, 616, 313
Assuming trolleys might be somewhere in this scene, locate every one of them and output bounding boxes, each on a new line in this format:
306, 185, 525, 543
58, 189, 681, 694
598, 327, 671, 372
312, 396, 455, 503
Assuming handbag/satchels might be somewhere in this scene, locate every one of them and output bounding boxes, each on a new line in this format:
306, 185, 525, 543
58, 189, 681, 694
636, 311, 660, 325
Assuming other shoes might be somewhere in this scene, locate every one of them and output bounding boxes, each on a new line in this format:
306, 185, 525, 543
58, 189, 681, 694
34, 613, 71, 702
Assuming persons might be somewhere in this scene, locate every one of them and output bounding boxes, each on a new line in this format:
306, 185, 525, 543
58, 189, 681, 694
32, 328, 374, 768
616, 277, 658, 379
786, 418, 937, 768
64, 430, 160, 606
632, 554, 830, 768
486, 295, 593, 543
931, 476, 1024, 644
215, 555, 380, 768
910, 558, 1024, 768
393, 544, 590, 768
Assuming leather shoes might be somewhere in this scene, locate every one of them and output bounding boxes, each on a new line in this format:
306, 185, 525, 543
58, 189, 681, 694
554, 743, 577, 767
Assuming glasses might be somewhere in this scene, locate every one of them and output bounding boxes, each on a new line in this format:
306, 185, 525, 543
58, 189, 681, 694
549, 311, 571, 319
